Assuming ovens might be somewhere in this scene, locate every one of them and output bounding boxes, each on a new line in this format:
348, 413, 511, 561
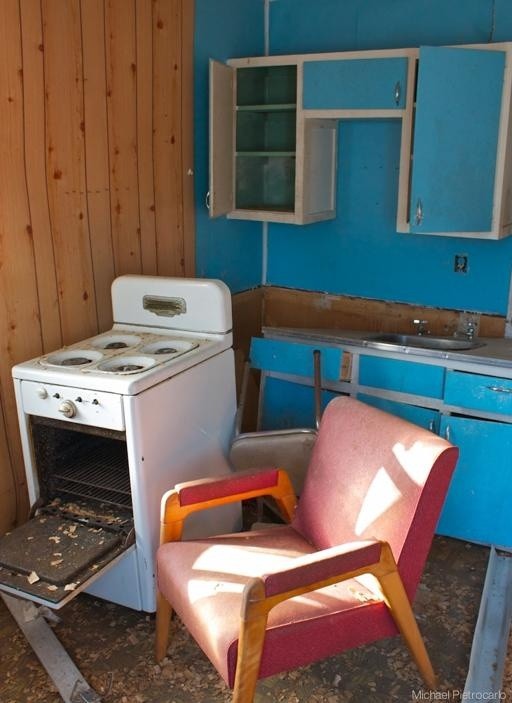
0, 413, 239, 614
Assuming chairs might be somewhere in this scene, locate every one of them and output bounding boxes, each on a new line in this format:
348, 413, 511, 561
155, 395, 459, 703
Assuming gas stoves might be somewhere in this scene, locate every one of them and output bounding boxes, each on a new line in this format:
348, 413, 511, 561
11, 330, 233, 432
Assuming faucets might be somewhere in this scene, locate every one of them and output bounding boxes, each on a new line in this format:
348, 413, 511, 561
412, 319, 433, 335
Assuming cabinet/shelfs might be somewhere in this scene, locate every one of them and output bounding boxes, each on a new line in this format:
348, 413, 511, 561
205, 55, 338, 226
302, 49, 409, 120
396, 41, 512, 240
251, 327, 512, 553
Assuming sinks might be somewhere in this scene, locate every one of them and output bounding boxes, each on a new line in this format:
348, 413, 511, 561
365, 333, 484, 353
